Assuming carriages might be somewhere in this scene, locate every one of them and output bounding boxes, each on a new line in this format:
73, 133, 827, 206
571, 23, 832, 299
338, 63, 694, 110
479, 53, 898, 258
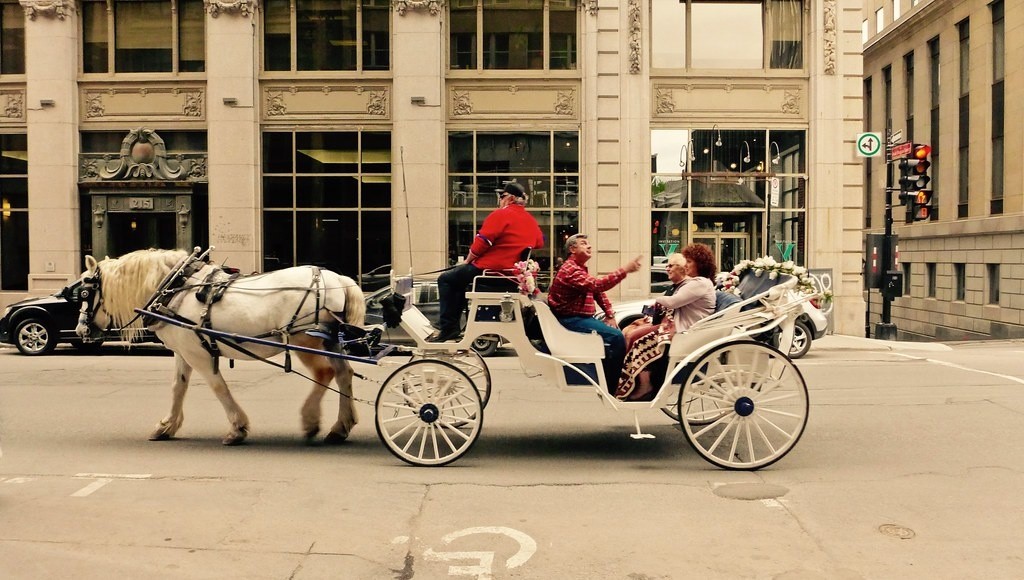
75, 245, 820, 471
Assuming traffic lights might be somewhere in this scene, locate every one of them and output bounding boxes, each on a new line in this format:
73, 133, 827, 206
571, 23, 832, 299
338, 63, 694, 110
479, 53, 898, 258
899, 144, 934, 223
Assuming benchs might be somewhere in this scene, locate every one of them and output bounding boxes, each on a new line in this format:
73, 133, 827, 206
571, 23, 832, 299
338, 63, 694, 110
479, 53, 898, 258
666, 289, 745, 374
471, 245, 533, 294
530, 293, 611, 394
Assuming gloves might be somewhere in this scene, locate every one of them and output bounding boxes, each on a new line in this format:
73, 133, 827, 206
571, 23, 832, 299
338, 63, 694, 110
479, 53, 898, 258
379, 293, 406, 328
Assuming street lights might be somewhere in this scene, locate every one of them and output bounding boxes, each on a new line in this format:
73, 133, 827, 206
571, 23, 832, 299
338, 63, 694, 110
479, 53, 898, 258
731, 141, 750, 179
757, 142, 780, 208
704, 124, 722, 172
679, 139, 695, 244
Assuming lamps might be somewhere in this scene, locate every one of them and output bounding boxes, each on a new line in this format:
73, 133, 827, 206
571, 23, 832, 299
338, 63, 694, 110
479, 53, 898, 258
769, 141, 780, 165
740, 140, 751, 162
711, 124, 723, 146
687, 138, 695, 162
679, 144, 686, 166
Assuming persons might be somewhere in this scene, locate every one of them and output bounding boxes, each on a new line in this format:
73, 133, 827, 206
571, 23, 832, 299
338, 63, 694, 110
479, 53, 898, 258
621, 244, 718, 400
427, 182, 545, 342
547, 234, 643, 397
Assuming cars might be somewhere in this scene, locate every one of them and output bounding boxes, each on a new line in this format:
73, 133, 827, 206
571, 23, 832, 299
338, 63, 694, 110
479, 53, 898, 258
355, 264, 391, 288
0, 266, 240, 356
591, 272, 828, 359
364, 279, 548, 357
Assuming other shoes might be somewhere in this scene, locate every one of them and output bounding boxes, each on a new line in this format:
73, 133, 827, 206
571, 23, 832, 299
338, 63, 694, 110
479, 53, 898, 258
425, 330, 461, 342
432, 320, 442, 329
630, 384, 652, 399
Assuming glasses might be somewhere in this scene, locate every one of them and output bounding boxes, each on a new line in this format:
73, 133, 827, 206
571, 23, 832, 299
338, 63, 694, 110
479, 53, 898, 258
666, 263, 679, 268
500, 193, 512, 200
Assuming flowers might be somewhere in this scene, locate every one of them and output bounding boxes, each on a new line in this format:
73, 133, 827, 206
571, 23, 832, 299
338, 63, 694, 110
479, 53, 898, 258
714, 255, 831, 309
515, 260, 544, 299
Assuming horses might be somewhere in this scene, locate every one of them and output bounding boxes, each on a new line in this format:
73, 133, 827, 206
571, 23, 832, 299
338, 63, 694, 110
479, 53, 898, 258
76, 249, 367, 446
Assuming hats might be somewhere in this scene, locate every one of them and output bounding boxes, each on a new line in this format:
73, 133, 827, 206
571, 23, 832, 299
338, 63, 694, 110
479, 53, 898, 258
495, 182, 524, 198
